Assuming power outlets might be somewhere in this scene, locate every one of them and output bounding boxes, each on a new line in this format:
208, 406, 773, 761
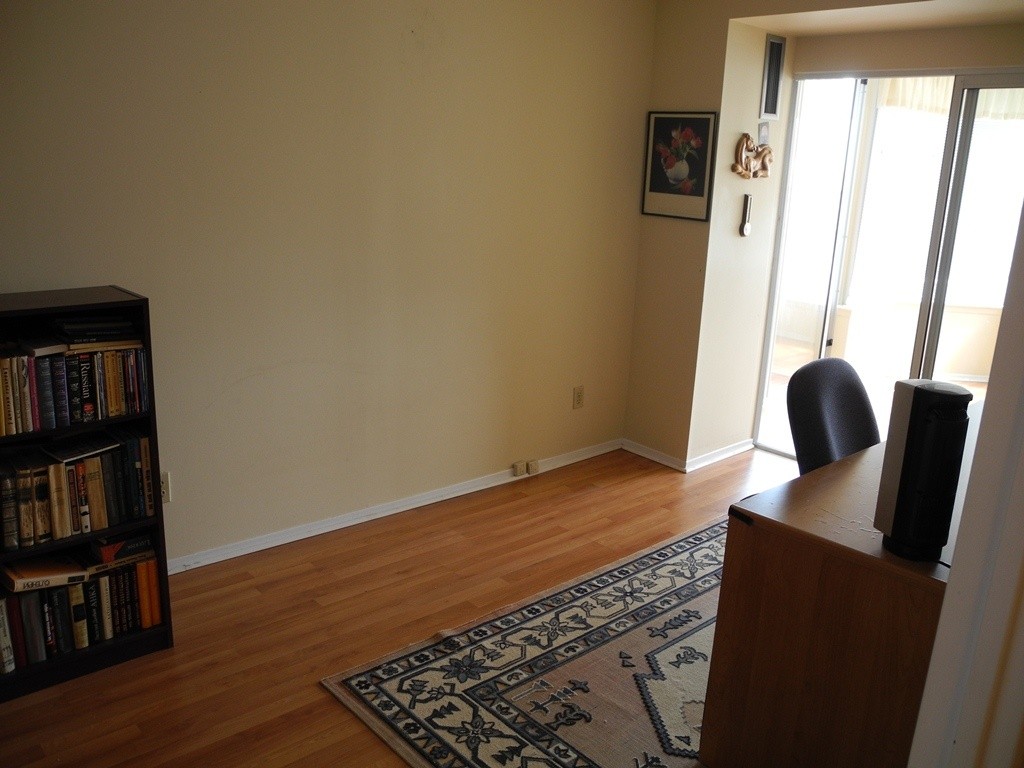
573, 385, 586, 408
159, 472, 171, 504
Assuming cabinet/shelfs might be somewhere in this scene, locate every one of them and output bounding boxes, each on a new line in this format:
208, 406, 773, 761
0, 284, 174, 706
698, 402, 984, 768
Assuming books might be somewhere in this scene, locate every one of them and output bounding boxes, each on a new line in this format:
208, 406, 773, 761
0, 317, 150, 437
0, 531, 161, 675
0, 427, 155, 551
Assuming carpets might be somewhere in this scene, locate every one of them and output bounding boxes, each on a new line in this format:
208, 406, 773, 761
320, 512, 729, 768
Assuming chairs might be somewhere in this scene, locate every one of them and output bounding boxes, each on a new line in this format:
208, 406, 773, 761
785, 357, 881, 475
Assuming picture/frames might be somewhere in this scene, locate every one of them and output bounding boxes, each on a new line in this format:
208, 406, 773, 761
642, 110, 719, 222
